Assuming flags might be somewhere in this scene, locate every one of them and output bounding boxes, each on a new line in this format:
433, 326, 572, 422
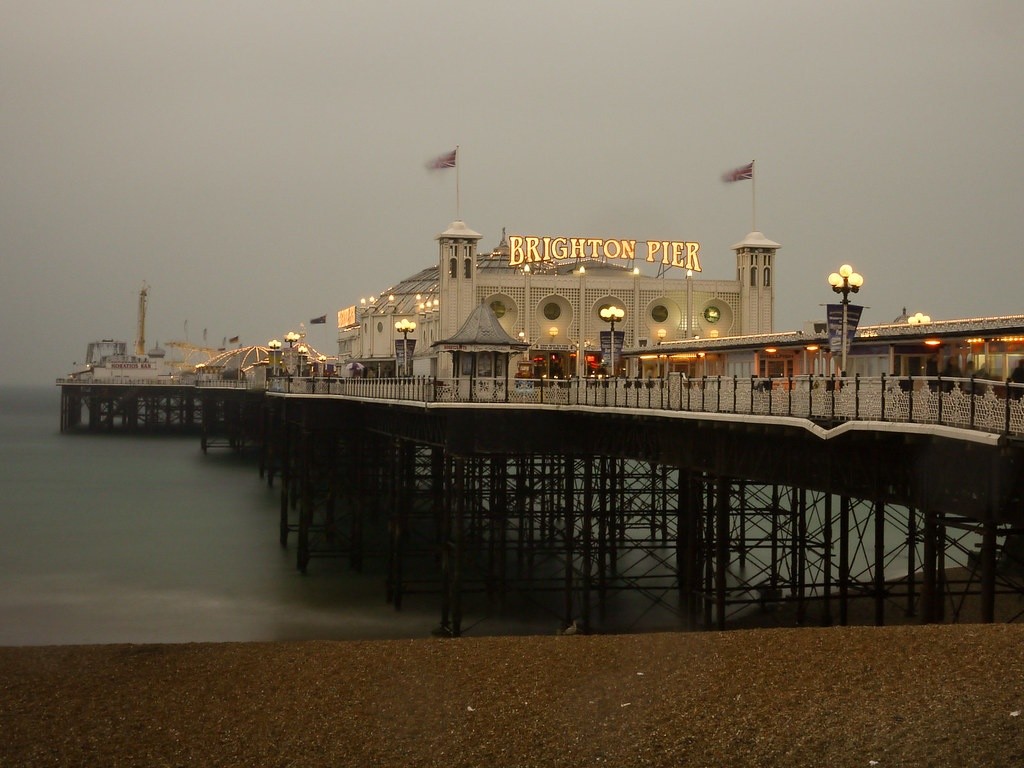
229, 336, 238, 344
310, 315, 326, 324
423, 149, 456, 169
721, 162, 752, 182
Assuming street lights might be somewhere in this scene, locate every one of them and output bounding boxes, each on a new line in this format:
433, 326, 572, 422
600, 305, 626, 378
318, 353, 326, 377
394, 318, 417, 375
285, 332, 301, 348
829, 263, 863, 376
295, 345, 308, 376
268, 339, 282, 376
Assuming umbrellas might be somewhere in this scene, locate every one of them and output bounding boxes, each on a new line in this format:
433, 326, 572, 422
346, 362, 365, 376
